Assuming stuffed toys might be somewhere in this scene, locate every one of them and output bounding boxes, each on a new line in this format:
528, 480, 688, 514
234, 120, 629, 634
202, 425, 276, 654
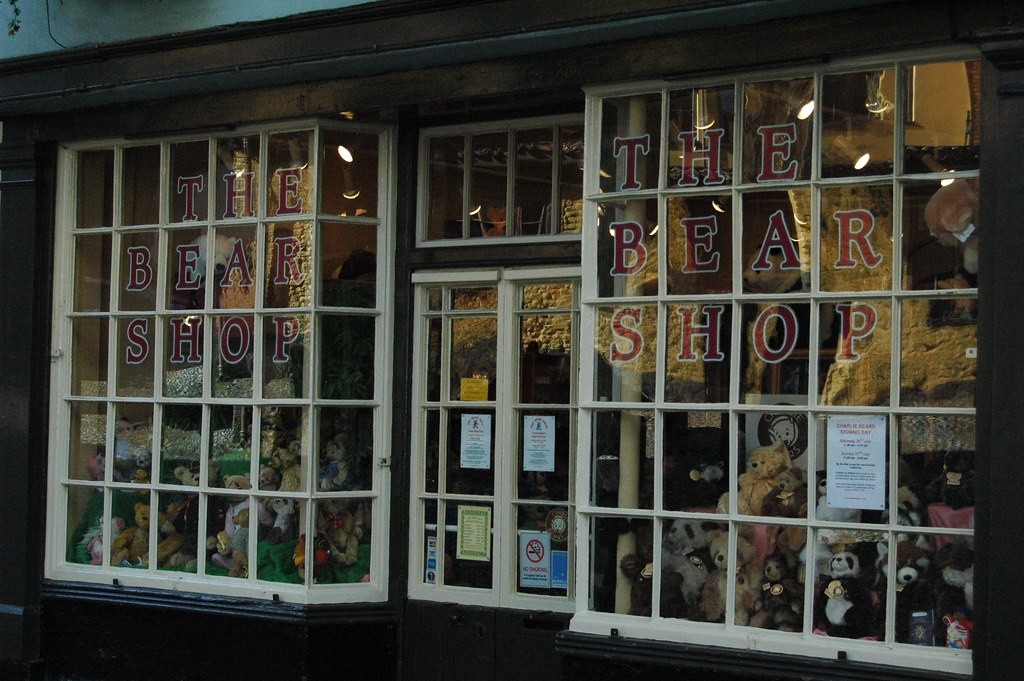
621, 440, 976, 649
924, 175, 981, 289
67, 432, 370, 585
184, 234, 237, 289
485, 206, 506, 237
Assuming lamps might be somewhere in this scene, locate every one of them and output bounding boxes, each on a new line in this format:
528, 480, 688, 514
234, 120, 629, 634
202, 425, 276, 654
711, 81, 955, 213
217, 133, 481, 215
